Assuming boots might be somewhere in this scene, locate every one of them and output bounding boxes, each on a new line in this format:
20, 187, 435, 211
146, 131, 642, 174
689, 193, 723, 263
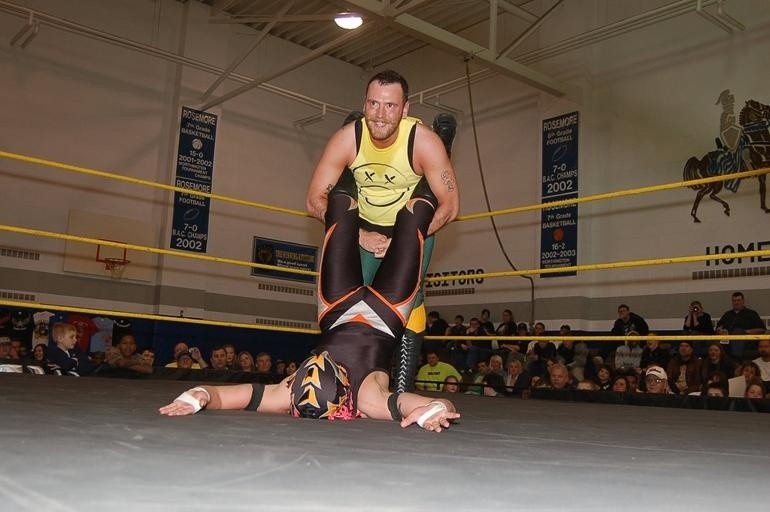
411, 115, 457, 206
329, 112, 363, 203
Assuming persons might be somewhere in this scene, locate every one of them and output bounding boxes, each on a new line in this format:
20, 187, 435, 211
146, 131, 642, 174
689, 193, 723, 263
0, 321, 299, 385
159, 112, 461, 432
417, 293, 770, 399
306, 71, 458, 393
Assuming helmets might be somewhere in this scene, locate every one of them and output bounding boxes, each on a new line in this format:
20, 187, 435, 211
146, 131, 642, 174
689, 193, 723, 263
285, 350, 350, 419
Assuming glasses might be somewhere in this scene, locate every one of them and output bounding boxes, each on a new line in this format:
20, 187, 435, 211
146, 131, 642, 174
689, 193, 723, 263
648, 378, 663, 383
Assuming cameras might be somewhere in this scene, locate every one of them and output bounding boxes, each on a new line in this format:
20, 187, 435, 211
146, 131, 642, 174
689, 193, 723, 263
694, 306, 699, 312
189, 348, 196, 353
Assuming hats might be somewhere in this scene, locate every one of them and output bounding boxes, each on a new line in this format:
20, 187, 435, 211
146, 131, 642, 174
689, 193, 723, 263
0, 337, 12, 345
646, 366, 667, 379
